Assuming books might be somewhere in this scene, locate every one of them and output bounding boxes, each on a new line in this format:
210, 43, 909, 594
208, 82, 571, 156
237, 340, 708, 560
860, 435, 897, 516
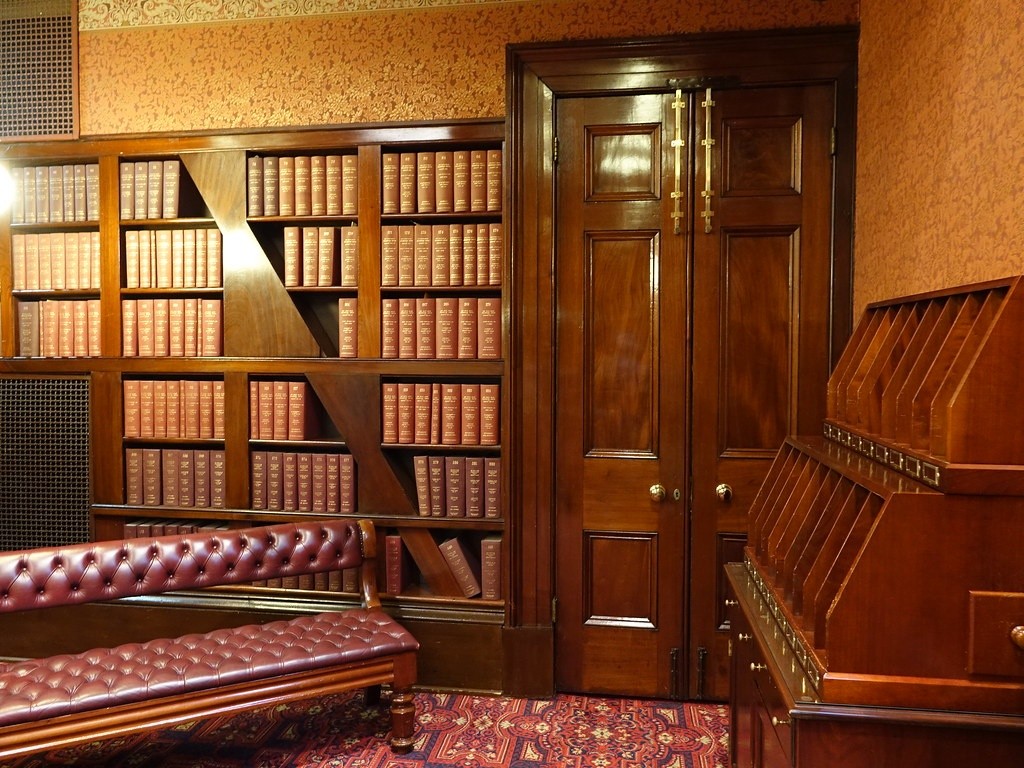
10, 151, 503, 601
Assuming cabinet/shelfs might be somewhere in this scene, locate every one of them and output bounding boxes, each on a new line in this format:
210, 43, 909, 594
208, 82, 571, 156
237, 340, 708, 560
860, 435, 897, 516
0, 117, 505, 698
718, 276, 1024, 768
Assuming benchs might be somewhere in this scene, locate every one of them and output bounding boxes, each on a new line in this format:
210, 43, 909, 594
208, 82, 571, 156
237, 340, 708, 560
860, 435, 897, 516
0, 519, 421, 768
